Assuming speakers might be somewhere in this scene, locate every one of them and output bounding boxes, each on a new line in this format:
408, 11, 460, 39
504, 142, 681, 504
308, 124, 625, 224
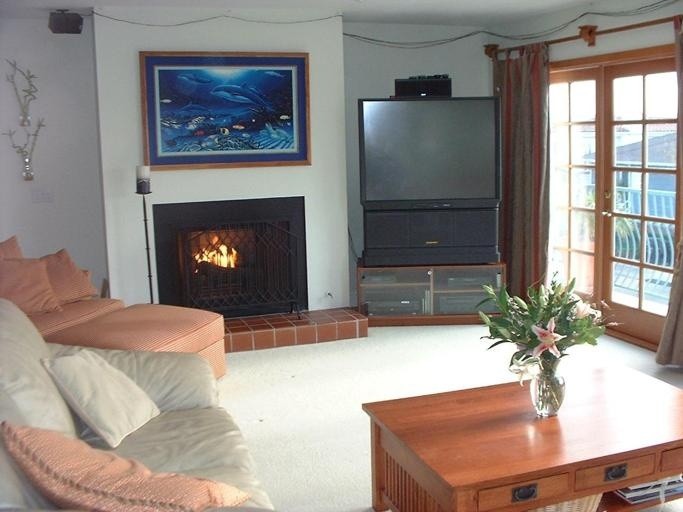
47, 10, 85, 35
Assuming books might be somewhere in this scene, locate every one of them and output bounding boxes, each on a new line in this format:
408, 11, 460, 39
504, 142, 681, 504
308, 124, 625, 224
612, 472, 683, 504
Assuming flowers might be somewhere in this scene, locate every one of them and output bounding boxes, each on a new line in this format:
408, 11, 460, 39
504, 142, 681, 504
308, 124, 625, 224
470, 273, 629, 388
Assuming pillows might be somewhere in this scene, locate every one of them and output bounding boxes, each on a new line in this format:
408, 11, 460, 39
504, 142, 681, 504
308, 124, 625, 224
0, 258, 56, 314
4, 415, 252, 510
39, 247, 99, 299
43, 346, 163, 449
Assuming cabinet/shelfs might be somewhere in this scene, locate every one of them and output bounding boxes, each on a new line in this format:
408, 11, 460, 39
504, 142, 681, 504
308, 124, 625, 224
356, 256, 507, 325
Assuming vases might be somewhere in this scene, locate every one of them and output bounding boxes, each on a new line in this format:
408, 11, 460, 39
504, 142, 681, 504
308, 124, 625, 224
530, 365, 567, 418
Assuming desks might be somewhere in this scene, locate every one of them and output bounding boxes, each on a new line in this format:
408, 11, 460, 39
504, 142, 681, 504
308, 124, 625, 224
360, 367, 683, 511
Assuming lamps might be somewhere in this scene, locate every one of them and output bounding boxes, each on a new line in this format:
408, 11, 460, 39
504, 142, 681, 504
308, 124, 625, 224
132, 166, 158, 304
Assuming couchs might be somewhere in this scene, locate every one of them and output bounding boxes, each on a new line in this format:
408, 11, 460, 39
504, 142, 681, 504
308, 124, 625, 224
0, 237, 226, 377
1, 304, 276, 511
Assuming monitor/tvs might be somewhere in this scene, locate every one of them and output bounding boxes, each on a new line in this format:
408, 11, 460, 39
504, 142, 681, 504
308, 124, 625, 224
357, 97, 504, 210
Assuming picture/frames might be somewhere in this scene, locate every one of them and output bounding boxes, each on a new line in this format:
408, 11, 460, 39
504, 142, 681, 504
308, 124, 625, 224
136, 48, 314, 169
